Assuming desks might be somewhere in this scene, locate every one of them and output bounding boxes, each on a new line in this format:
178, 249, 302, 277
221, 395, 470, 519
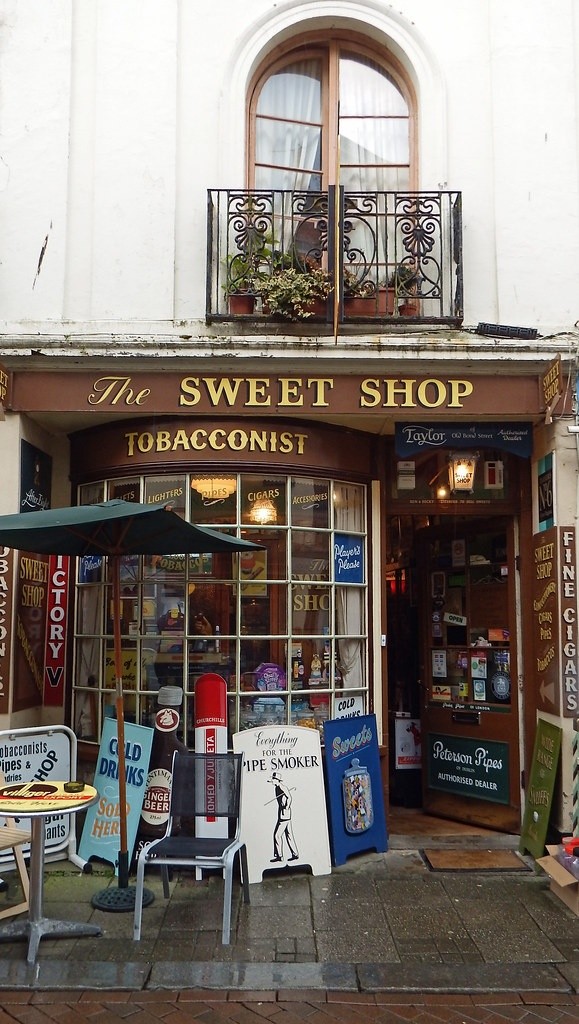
0, 781, 103, 966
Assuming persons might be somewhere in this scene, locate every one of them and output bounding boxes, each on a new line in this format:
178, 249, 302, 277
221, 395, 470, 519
310, 655, 322, 678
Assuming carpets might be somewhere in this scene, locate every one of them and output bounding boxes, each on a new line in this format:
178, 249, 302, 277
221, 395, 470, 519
418, 849, 532, 872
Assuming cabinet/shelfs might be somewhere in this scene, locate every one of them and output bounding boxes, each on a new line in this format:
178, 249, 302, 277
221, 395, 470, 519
418, 516, 521, 835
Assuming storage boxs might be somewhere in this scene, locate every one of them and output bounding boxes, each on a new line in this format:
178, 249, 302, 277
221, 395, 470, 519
535, 845, 579, 917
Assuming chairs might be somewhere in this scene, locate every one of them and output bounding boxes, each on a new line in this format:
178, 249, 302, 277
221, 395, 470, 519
132, 749, 250, 944
0, 771, 31, 919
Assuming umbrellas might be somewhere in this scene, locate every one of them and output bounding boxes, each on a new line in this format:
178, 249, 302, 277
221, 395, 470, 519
0, 498, 267, 889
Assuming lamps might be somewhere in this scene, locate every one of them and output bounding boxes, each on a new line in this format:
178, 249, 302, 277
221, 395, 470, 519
249, 479, 276, 525
191, 474, 237, 498
448, 449, 479, 495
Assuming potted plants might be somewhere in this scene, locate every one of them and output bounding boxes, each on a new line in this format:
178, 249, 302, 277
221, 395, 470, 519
225, 226, 424, 324
